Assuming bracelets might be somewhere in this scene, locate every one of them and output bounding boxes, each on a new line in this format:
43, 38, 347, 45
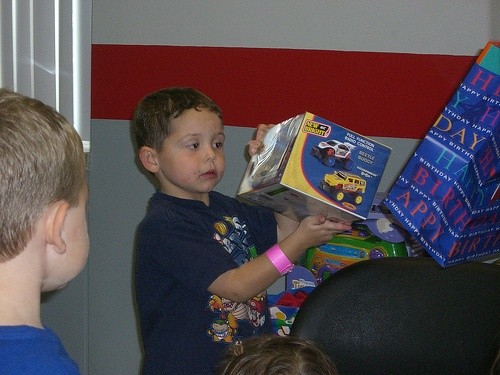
265, 244, 294, 277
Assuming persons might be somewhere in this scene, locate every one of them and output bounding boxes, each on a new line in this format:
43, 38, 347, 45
221, 336, 338, 375
131, 88, 353, 375
0, 88, 91, 375
